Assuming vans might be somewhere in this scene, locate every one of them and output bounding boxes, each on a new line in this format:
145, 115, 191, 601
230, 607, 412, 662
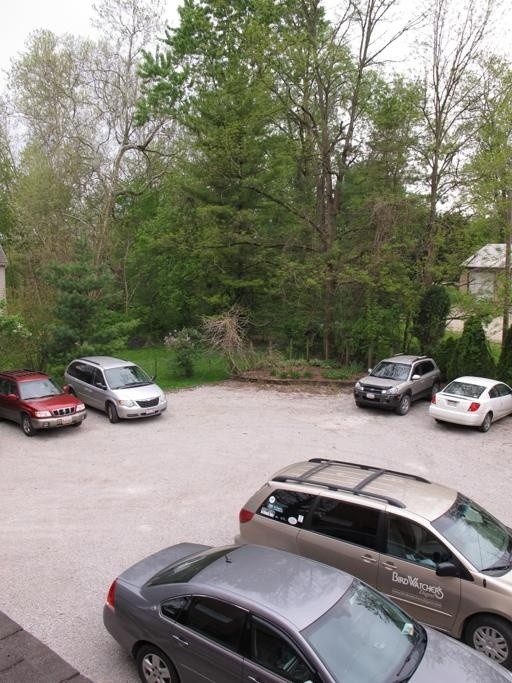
64, 354, 168, 424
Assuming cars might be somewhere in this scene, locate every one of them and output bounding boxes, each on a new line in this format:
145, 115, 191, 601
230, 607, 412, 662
428, 373, 511, 432
102, 537, 511, 682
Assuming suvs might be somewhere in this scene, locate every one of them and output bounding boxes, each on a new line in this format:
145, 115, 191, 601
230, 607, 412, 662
353, 351, 441, 415
234, 456, 509, 672
0, 368, 88, 437
